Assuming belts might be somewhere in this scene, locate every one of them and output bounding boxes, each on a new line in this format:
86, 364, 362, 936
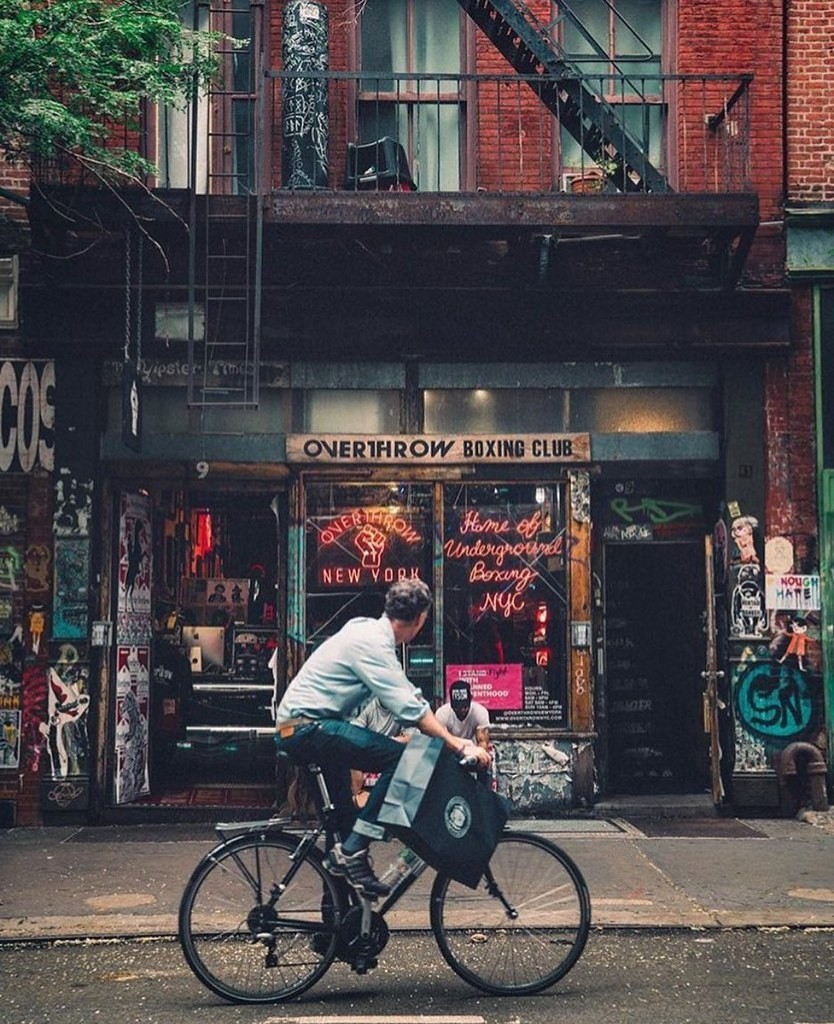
276, 718, 312, 732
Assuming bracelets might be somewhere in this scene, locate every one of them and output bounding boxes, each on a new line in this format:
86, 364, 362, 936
458, 745, 465, 755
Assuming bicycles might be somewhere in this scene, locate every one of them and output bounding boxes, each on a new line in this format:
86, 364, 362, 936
176, 749, 593, 1007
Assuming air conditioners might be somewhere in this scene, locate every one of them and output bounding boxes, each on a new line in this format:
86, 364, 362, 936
559, 173, 583, 193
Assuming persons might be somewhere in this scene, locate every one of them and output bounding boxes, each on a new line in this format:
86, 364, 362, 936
349, 680, 490, 809
273, 576, 493, 969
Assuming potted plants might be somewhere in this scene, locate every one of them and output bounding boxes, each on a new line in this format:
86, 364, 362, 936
570, 158, 618, 193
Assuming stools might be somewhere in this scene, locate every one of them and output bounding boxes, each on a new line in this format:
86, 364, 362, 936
343, 135, 418, 192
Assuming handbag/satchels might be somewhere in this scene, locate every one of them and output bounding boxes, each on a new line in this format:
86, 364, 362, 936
375, 732, 512, 889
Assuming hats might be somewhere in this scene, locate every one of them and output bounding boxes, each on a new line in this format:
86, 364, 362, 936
450, 681, 471, 706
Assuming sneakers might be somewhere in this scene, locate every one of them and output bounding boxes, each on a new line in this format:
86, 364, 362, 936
322, 842, 391, 896
309, 933, 378, 969
